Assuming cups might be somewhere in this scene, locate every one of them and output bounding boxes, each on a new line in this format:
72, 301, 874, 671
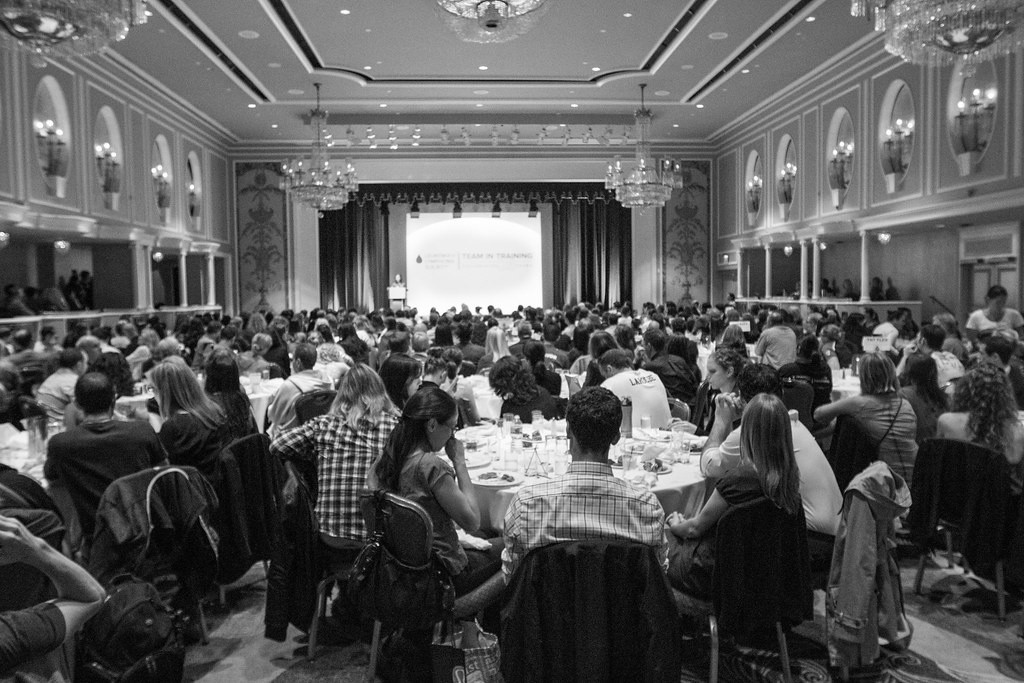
493, 411, 694, 471
262, 369, 270, 381
250, 373, 261, 392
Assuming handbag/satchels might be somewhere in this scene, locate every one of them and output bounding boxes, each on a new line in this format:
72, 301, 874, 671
338, 491, 455, 630
375, 626, 431, 683
432, 620, 506, 683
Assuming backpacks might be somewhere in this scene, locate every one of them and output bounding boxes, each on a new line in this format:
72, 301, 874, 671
75, 573, 185, 683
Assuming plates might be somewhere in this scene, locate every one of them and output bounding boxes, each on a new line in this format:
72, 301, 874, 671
440, 426, 700, 487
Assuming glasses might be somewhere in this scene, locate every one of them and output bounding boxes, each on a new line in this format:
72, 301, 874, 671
430, 420, 458, 435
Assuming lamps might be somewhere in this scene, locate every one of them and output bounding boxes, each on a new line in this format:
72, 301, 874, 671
279, 82, 360, 210
884, 118, 913, 195
528, 200, 539, 213
94, 142, 123, 209
828, 140, 853, 209
380, 201, 390, 215
151, 163, 176, 227
748, 175, 762, 226
0, 0, 150, 57
954, 85, 997, 176
410, 201, 419, 214
452, 202, 463, 212
776, 162, 796, 222
439, 0, 552, 41
32, 119, 69, 197
850, 1, 1024, 78
603, 84, 684, 210
492, 200, 502, 214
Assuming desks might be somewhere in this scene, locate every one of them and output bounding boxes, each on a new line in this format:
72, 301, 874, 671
462, 375, 506, 416
444, 418, 710, 542
196, 375, 284, 435
0, 427, 59, 491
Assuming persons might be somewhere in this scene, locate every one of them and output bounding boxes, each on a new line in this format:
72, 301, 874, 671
0, 268, 95, 320
0, 295, 1024, 683
391, 273, 407, 287
965, 286, 1024, 351
793, 277, 886, 301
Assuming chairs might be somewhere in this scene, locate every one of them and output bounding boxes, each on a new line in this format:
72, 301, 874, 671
687, 502, 802, 683
94, 464, 211, 647
830, 412, 876, 485
779, 376, 837, 449
295, 389, 335, 423
357, 491, 474, 682
281, 459, 354, 660
495, 541, 680, 683
909, 436, 1019, 627
16, 396, 50, 425
668, 377, 709, 426
809, 462, 905, 683
207, 435, 276, 610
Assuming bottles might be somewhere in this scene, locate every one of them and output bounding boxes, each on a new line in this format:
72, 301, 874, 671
510, 415, 522, 455
621, 395, 632, 438
